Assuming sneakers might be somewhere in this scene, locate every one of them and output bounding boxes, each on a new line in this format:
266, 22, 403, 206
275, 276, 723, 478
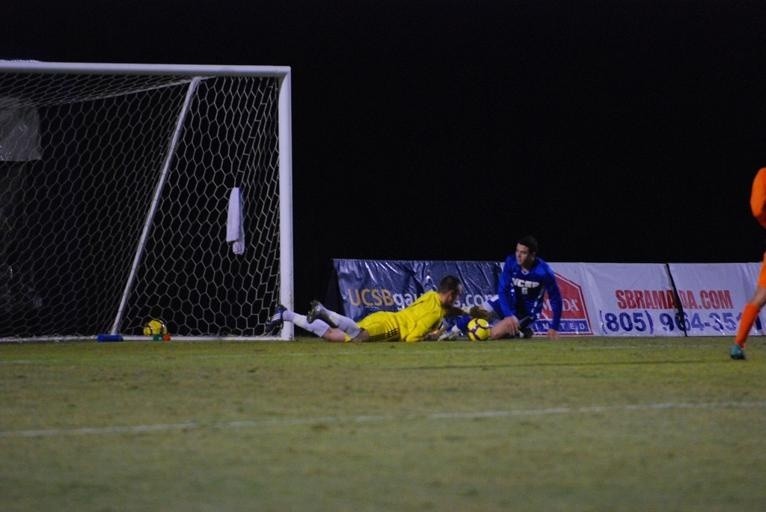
307, 300, 321, 323
731, 345, 744, 359
271, 305, 286, 335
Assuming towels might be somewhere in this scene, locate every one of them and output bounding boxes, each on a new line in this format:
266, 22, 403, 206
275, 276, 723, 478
226, 187, 246, 256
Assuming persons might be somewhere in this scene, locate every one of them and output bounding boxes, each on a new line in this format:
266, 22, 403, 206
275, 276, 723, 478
266, 278, 491, 341
443, 234, 561, 340
732, 165, 766, 359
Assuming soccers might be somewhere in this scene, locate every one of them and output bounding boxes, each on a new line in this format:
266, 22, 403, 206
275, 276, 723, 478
143, 319, 167, 336
467, 318, 490, 341
